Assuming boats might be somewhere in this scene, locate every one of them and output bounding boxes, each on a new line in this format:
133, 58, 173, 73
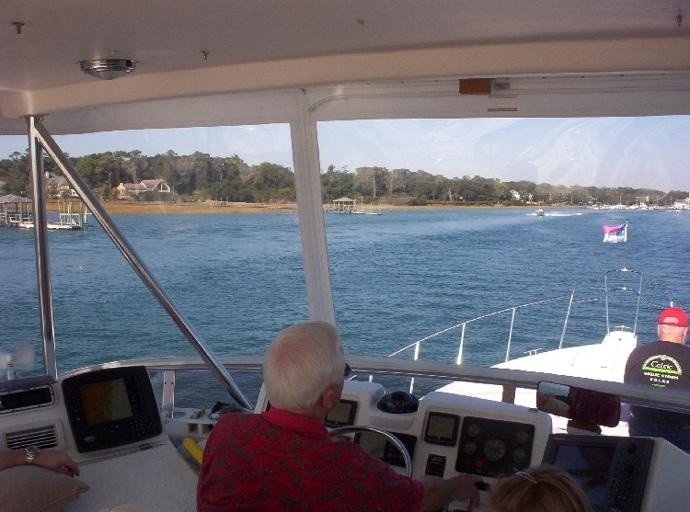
600, 218, 629, 243
536, 207, 544, 217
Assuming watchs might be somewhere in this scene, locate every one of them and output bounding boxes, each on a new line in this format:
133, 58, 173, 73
23, 445, 40, 465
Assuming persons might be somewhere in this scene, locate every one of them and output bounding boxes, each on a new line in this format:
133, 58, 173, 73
0, 445, 81, 478
196, 319, 485, 511
623, 306, 689, 452
489, 464, 595, 511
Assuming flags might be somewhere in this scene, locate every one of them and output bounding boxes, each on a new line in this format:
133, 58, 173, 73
601, 221, 630, 244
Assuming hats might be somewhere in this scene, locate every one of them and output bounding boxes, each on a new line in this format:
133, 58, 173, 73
659, 306, 690, 328
